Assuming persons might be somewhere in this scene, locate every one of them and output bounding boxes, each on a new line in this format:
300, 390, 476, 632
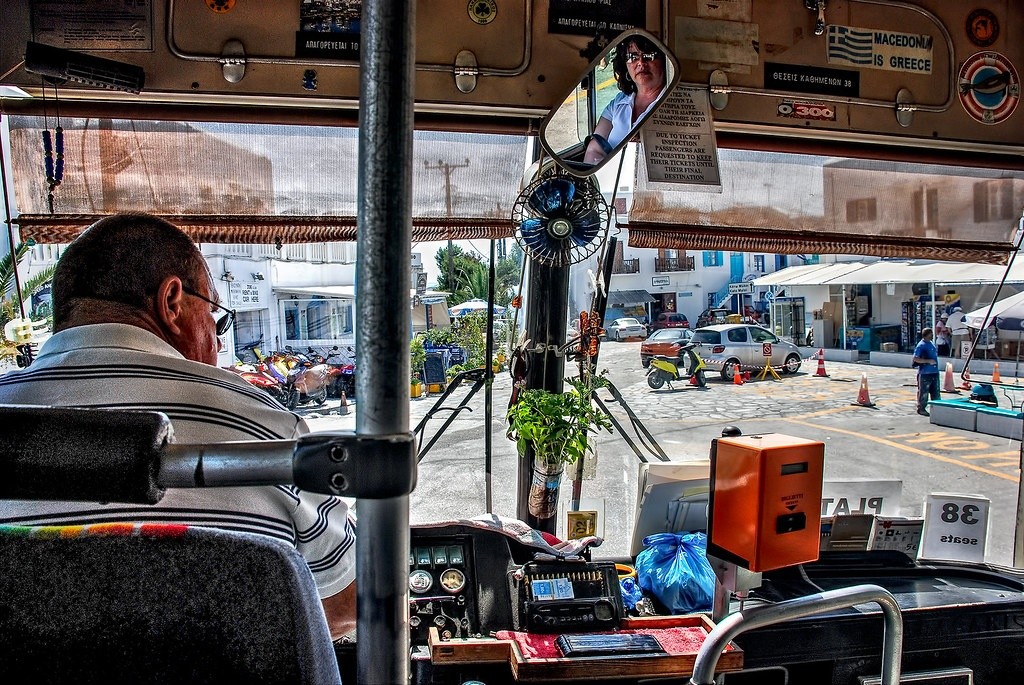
913, 328, 940, 417
945, 307, 973, 359
936, 313, 950, 356
0, 211, 358, 642
583, 34, 666, 166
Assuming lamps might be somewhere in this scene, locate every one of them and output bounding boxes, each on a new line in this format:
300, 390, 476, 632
220, 274, 229, 281
251, 272, 264, 280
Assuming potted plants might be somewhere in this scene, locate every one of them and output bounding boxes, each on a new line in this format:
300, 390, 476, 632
411, 313, 614, 516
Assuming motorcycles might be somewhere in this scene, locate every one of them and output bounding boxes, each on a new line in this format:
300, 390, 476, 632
234, 333, 356, 410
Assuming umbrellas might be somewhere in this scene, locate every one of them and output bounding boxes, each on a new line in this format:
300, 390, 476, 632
448, 297, 513, 326
960, 290, 1024, 377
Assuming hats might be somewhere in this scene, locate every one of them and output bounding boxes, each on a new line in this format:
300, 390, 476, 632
941, 313, 949, 318
954, 307, 963, 311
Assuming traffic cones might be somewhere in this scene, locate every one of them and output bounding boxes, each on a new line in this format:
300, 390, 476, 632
733, 365, 743, 385
812, 349, 830, 377
991, 363, 1003, 383
340, 391, 347, 406
851, 372, 876, 406
940, 361, 961, 393
961, 367, 972, 390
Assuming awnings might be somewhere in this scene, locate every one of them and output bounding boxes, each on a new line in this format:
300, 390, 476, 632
272, 285, 454, 303
608, 290, 656, 304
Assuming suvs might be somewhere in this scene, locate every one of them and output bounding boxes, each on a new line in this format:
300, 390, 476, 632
696, 308, 731, 328
649, 313, 690, 334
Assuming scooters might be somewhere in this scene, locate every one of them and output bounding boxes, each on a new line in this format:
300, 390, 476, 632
644, 342, 707, 391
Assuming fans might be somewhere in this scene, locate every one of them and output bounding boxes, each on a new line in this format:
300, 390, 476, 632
511, 176, 609, 267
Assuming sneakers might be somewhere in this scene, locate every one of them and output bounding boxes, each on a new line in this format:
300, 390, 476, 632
917, 409, 930, 416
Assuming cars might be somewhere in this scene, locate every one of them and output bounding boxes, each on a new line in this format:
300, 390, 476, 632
778, 312, 815, 345
641, 328, 694, 368
605, 317, 647, 342
686, 324, 802, 381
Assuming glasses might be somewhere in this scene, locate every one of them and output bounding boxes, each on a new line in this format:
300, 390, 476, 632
625, 52, 658, 63
182, 284, 236, 335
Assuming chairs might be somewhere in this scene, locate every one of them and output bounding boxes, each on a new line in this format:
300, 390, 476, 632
0, 522, 342, 685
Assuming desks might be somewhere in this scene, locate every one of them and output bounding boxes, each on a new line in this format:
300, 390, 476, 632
1000, 387, 1024, 412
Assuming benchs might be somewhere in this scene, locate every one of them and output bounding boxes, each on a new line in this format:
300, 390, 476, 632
976, 407, 1024, 441
926, 398, 987, 431
